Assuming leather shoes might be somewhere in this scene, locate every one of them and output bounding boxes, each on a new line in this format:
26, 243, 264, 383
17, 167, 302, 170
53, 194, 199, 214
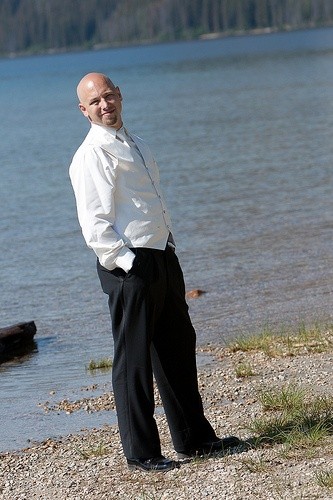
126, 450, 181, 472
178, 435, 241, 456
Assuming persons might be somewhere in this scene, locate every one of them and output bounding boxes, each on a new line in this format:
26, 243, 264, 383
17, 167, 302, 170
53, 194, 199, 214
68, 72, 241, 472
